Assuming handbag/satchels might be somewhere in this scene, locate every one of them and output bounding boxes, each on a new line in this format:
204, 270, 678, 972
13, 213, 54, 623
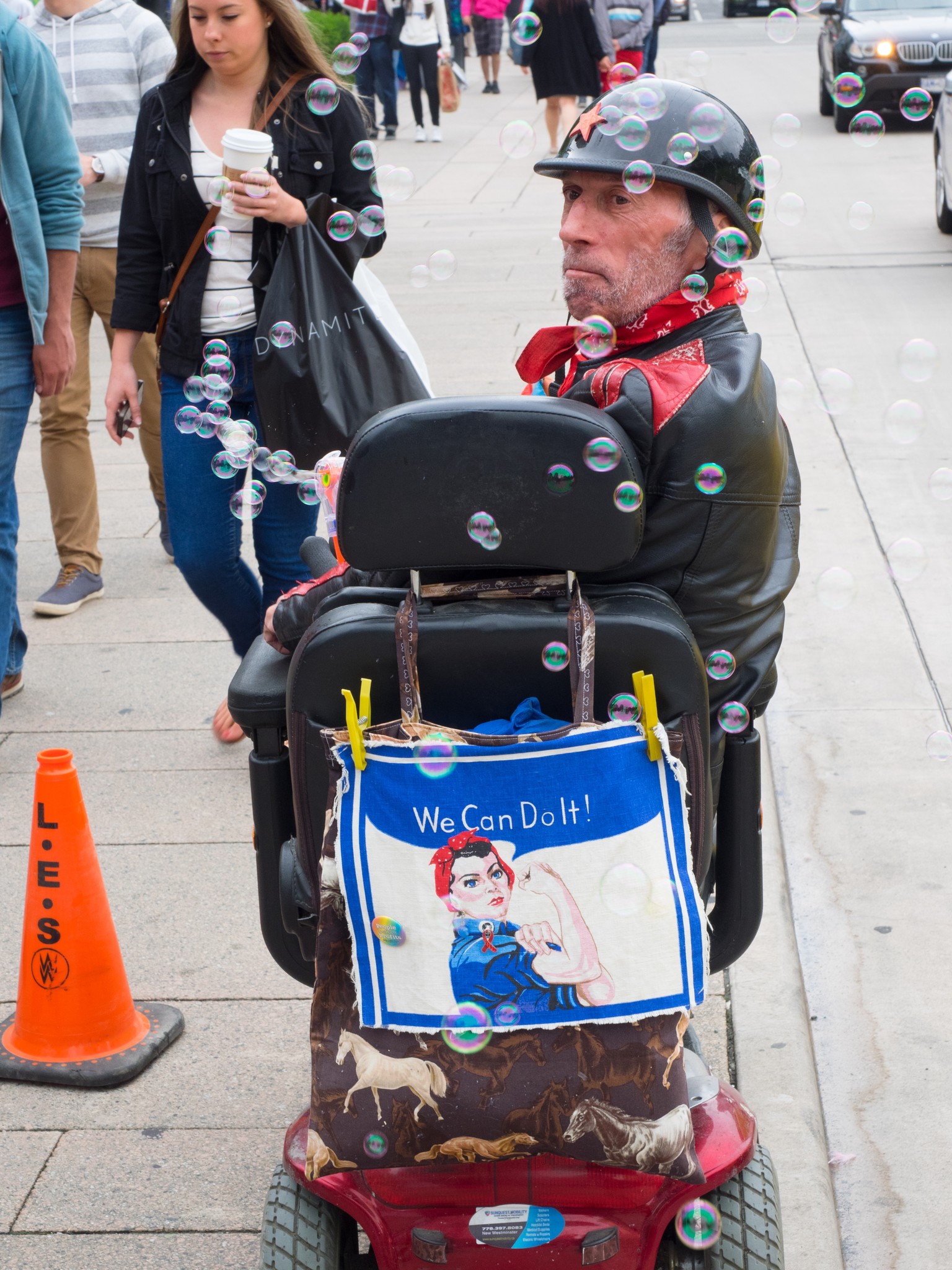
448, 58, 470, 90
256, 193, 436, 475
437, 58, 461, 116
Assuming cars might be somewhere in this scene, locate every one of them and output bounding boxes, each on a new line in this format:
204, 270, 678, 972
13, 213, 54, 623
927, 69, 952, 232
664, 0, 837, 20
815, 0, 950, 135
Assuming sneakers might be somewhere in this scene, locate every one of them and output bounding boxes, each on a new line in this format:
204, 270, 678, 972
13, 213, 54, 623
366, 129, 377, 141
415, 125, 425, 143
492, 80, 499, 94
431, 126, 443, 143
384, 129, 395, 141
483, 82, 491, 94
34, 564, 102, 617
159, 507, 175, 559
0, 669, 23, 702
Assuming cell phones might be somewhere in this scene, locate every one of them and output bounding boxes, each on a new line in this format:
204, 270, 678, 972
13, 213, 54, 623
117, 379, 145, 438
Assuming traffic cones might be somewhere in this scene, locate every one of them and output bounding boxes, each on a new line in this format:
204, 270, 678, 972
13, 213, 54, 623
0, 743, 186, 1092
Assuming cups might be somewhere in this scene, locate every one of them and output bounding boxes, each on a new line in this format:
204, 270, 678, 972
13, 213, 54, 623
220, 128, 274, 219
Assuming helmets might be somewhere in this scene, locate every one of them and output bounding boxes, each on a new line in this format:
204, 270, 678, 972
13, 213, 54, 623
531, 75, 767, 259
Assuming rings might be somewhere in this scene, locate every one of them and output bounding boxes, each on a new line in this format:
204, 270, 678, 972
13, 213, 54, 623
264, 186, 270, 197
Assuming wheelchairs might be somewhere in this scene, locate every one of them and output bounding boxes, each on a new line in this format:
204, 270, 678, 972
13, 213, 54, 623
225, 395, 793, 1270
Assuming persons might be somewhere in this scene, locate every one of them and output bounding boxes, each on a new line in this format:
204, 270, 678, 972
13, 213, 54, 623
261, 75, 804, 744
1, 0, 88, 702
312, 0, 671, 160
103, 0, 387, 746
14, 0, 179, 619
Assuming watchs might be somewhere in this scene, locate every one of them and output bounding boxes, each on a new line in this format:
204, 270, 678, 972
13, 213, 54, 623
92, 155, 105, 183
